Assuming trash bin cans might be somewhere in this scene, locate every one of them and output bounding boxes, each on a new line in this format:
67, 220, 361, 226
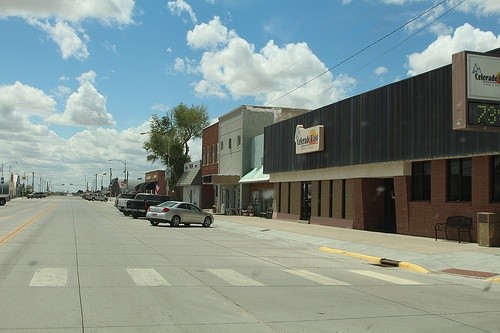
477, 212, 496, 247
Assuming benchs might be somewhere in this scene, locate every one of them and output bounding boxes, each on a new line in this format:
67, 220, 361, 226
260, 209, 273, 219
435, 216, 473, 244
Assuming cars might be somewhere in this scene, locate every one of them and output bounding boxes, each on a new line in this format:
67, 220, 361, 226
146, 201, 214, 227
80, 192, 94, 201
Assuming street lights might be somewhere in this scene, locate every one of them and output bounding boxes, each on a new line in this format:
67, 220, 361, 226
1, 161, 18, 183
108, 159, 126, 179
140, 132, 171, 195
100, 169, 112, 184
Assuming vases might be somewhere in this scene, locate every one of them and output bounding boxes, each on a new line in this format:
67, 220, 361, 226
244, 212, 248, 215
249, 213, 254, 216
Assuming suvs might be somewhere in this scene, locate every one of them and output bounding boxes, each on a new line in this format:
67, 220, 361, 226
27, 192, 42, 199
118, 194, 136, 216
114, 194, 123, 212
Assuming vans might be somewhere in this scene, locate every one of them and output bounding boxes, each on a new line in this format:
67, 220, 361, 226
0, 183, 10, 206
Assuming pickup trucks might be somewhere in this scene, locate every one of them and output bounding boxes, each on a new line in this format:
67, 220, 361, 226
127, 195, 176, 219
86, 193, 108, 202
40, 192, 47, 198
